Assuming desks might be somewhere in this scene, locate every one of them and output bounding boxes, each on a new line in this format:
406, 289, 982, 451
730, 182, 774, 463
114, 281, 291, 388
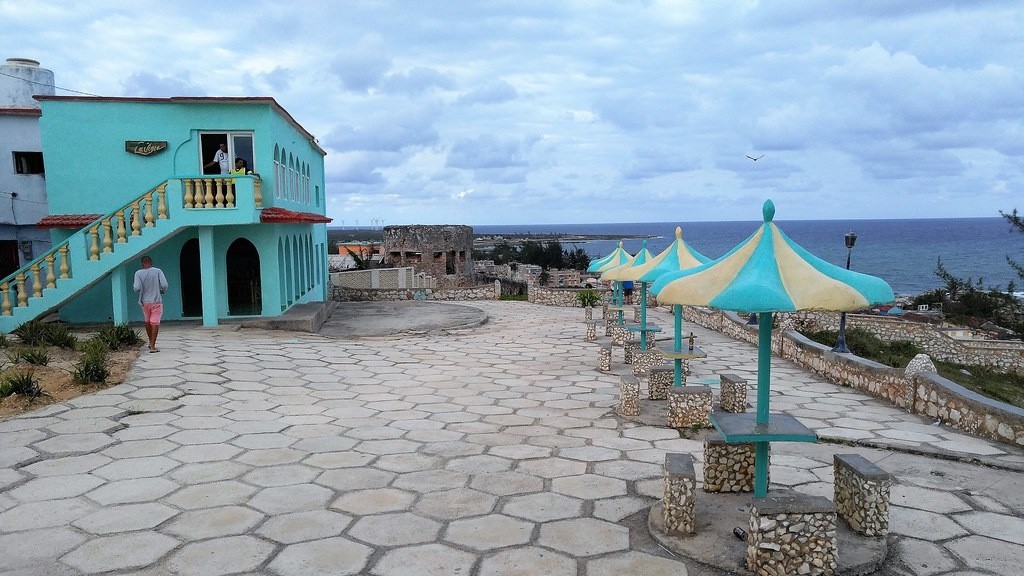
605, 297, 623, 305
607, 307, 634, 325
624, 324, 662, 349
708, 411, 817, 496
657, 346, 707, 387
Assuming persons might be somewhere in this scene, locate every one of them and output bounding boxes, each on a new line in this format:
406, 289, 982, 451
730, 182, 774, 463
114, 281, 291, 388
133, 256, 168, 353
203, 140, 251, 175
623, 281, 633, 304
602, 280, 606, 285
598, 276, 601, 279
594, 275, 598, 279
608, 280, 614, 292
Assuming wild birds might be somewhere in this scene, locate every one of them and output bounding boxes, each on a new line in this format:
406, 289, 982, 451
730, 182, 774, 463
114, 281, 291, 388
744, 154, 766, 162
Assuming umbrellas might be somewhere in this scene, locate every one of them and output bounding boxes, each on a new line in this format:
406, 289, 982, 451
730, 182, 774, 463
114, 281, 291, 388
586, 199, 895, 424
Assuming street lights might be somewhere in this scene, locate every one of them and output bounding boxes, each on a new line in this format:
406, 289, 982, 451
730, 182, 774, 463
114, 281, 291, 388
830, 228, 858, 353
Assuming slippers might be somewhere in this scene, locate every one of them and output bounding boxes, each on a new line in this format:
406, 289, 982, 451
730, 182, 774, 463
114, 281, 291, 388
148, 344, 151, 348
149, 348, 160, 353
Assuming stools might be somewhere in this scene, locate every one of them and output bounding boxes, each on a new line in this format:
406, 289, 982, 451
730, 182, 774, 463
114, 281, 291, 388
833, 453, 890, 537
634, 306, 642, 323
605, 319, 626, 336
600, 343, 612, 371
704, 436, 772, 493
680, 359, 691, 376
647, 366, 686, 400
587, 320, 597, 341
665, 386, 714, 428
664, 452, 696, 536
585, 306, 592, 323
624, 339, 652, 364
620, 375, 641, 416
631, 349, 663, 376
747, 495, 837, 576
611, 326, 634, 345
646, 322, 655, 347
720, 374, 748, 413
603, 304, 609, 319
